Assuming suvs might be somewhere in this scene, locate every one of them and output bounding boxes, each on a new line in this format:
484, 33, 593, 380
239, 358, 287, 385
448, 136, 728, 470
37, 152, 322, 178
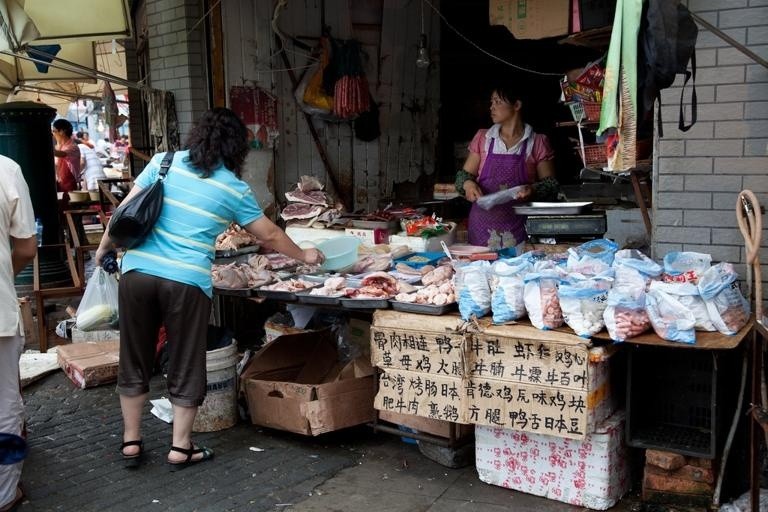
84, 223, 107, 245
190, 339, 240, 435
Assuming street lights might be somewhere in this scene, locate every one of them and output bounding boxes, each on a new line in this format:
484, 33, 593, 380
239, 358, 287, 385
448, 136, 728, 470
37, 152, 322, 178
107, 150, 175, 250
638, 0, 699, 138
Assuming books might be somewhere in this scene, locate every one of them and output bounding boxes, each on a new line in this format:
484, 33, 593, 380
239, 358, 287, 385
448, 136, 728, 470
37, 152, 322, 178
473, 345, 631, 511
57, 339, 119, 390
241, 331, 377, 438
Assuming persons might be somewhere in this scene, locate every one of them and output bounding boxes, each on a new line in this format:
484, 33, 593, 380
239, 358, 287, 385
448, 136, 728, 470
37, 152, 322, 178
93, 107, 326, 472
0, 155, 38, 510
453, 82, 560, 247
51, 118, 131, 191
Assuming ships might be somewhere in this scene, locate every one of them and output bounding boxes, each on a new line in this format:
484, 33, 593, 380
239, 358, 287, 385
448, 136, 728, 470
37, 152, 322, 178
613, 306, 649, 339
333, 75, 371, 119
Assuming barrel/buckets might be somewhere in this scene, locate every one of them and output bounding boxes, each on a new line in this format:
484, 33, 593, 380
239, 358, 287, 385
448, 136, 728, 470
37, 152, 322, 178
0, 479, 25, 512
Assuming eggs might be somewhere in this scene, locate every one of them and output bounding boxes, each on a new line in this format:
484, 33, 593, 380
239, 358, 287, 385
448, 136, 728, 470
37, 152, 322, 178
77, 304, 120, 330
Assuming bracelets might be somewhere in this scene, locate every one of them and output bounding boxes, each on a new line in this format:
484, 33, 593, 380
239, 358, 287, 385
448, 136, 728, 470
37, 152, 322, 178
67, 189, 100, 202
314, 235, 360, 271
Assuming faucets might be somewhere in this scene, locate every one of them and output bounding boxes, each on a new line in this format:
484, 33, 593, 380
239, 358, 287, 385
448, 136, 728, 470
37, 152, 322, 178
165, 443, 215, 473
119, 439, 145, 468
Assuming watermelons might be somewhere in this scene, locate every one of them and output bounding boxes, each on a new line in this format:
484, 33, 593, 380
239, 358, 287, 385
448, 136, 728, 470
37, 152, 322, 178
513, 201, 606, 243
